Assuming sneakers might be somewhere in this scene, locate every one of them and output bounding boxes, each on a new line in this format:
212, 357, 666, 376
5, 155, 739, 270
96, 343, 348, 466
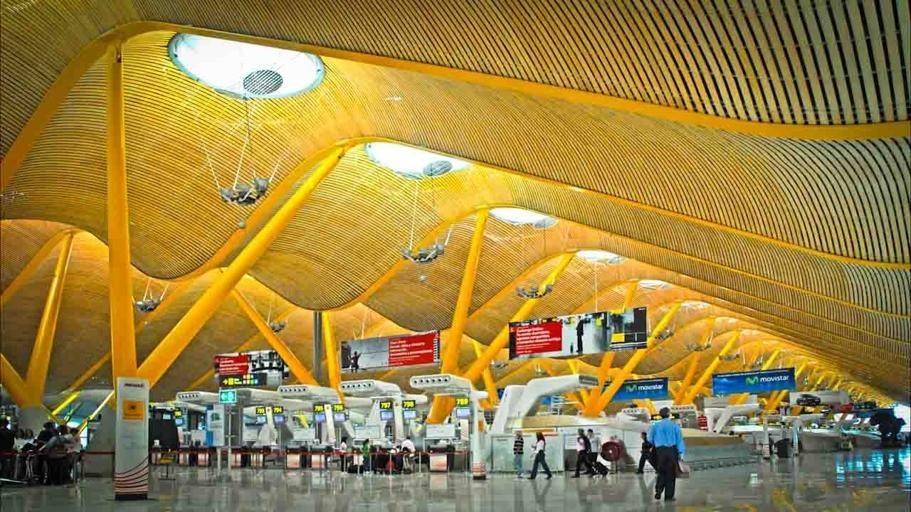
513, 474, 525, 479
588, 472, 597, 477
584, 469, 592, 475
544, 475, 552, 479
664, 496, 677, 502
570, 474, 580, 478
526, 477, 535, 480
655, 493, 661, 500
636, 470, 644, 475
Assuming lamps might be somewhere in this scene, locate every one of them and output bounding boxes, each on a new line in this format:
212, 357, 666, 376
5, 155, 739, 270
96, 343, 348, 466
366, 142, 470, 284
129, 265, 171, 313
167, 32, 326, 230
267, 287, 288, 333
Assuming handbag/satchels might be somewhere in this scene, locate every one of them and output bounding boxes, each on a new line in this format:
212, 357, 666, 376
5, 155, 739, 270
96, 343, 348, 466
674, 459, 690, 479
537, 451, 545, 462
585, 452, 598, 464
348, 465, 363, 474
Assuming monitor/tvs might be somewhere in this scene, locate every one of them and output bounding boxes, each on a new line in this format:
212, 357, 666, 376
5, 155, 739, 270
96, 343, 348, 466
333, 413, 345, 422
456, 408, 471, 419
380, 411, 393, 420
162, 413, 172, 421
174, 418, 185, 426
274, 415, 285, 425
256, 415, 266, 423
314, 414, 326, 423
403, 410, 416, 421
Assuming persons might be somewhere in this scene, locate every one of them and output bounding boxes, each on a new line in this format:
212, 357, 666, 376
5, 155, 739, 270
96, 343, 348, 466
645, 407, 685, 502
362, 438, 371, 474
634, 432, 658, 474
570, 429, 596, 478
1, 418, 85, 487
400, 436, 417, 474
339, 436, 349, 475
580, 429, 601, 475
347, 351, 361, 373
512, 430, 525, 479
575, 315, 583, 352
527, 431, 553, 481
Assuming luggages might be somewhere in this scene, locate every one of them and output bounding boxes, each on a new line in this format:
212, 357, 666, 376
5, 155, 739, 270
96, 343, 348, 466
588, 457, 609, 476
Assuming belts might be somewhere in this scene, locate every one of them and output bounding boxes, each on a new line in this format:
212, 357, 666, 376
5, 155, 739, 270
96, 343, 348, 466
656, 445, 676, 449
